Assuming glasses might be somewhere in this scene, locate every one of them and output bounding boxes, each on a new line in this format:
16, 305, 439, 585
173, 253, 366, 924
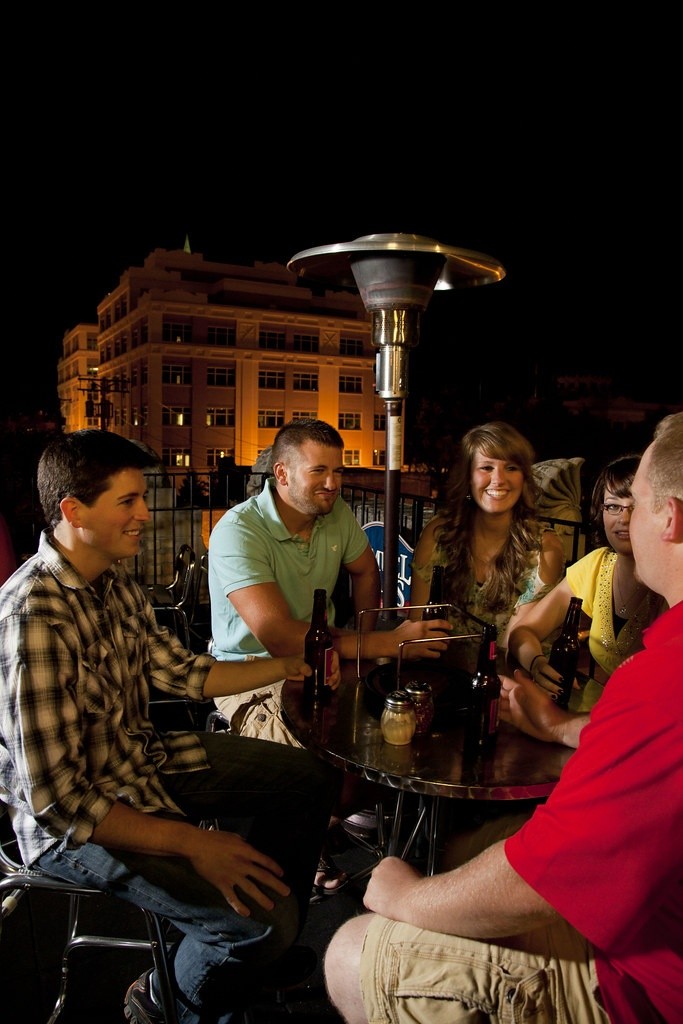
600, 504, 634, 515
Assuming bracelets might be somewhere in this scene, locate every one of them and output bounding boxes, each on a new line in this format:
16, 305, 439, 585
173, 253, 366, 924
530, 655, 545, 671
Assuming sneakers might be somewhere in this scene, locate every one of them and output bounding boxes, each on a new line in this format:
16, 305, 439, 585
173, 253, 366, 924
231, 945, 322, 1005
124, 967, 166, 1024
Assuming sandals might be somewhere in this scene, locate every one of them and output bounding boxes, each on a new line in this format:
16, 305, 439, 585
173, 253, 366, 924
311, 848, 350, 894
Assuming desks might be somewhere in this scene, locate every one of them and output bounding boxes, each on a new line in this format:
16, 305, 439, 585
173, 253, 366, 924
281, 644, 576, 904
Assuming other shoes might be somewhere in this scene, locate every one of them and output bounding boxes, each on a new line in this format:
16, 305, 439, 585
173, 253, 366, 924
341, 809, 410, 838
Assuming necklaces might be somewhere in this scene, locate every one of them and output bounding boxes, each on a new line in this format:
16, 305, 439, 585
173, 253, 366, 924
617, 566, 640, 614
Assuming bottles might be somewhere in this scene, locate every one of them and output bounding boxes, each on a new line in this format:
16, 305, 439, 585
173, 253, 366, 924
302, 589, 334, 697
422, 565, 449, 620
462, 624, 501, 759
405, 680, 435, 734
546, 597, 582, 710
380, 689, 417, 745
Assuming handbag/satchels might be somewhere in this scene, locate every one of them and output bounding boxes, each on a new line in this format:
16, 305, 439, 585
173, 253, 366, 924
104, 813, 230, 907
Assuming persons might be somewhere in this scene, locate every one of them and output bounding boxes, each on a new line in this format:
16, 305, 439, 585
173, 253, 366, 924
409, 423, 566, 656
209, 418, 454, 748
325, 413, 683, 1024
0, 427, 340, 1024
507, 455, 670, 702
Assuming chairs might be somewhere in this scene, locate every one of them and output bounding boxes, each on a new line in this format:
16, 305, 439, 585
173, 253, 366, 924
0, 543, 384, 1024
